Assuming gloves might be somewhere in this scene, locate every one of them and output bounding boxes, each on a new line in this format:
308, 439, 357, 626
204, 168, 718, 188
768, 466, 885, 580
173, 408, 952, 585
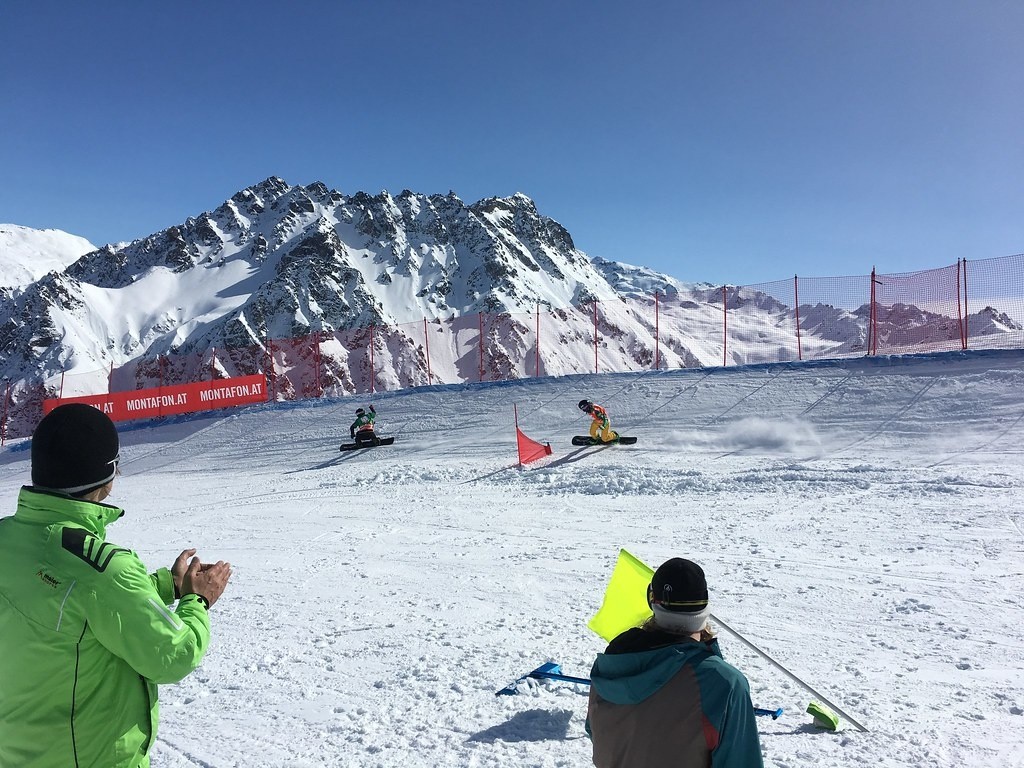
369, 405, 376, 412
350, 430, 355, 438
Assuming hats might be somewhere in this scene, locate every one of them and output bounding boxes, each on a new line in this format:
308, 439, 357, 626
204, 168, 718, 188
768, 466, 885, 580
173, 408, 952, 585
31, 403, 119, 497
647, 558, 708, 613
356, 408, 365, 417
578, 400, 589, 409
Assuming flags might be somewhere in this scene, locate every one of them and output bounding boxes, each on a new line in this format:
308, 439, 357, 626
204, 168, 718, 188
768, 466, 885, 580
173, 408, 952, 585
586, 551, 665, 644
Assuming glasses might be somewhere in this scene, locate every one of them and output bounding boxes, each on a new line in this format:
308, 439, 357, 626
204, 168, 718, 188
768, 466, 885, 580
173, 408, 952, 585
104, 455, 120, 467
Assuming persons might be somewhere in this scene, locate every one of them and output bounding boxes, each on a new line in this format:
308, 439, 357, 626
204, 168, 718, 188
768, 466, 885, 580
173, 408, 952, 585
577, 399, 619, 442
350, 406, 376, 442
584, 557, 763, 768
0, 404, 231, 768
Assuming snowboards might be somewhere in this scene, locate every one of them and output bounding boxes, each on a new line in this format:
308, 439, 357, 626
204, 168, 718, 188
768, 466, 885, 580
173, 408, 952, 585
569, 434, 639, 448
338, 434, 396, 454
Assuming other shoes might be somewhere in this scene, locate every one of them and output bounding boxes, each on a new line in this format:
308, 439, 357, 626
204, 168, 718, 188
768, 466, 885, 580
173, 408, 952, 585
354, 442, 362, 447
372, 439, 380, 446
611, 431, 619, 445
589, 434, 600, 445
